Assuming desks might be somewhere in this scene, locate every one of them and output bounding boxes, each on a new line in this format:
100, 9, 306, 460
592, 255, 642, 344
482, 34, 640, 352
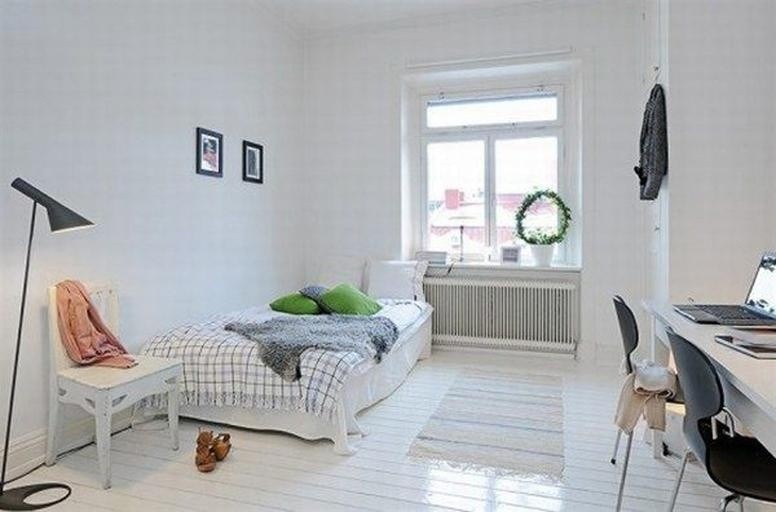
638, 299, 775, 459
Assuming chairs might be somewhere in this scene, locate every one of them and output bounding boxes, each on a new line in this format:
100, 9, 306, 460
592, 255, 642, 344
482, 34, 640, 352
49, 279, 183, 489
665, 326, 775, 512
611, 295, 687, 512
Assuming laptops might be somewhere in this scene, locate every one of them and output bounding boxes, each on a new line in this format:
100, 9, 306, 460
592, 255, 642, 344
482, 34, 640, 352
673, 253, 776, 328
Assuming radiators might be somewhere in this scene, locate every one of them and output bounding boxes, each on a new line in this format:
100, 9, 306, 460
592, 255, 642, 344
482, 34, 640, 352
422, 277, 577, 353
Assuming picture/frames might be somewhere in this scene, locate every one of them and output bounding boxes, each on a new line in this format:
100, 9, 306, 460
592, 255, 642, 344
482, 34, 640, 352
195, 127, 222, 178
242, 140, 262, 184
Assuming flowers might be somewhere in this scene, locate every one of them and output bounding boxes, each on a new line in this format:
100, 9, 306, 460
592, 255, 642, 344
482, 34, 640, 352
513, 186, 574, 247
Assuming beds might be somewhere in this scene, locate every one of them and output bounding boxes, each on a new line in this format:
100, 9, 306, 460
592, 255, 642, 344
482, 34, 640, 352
130, 259, 434, 457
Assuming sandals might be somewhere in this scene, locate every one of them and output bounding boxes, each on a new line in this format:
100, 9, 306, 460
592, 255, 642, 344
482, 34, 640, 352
195, 430, 231, 471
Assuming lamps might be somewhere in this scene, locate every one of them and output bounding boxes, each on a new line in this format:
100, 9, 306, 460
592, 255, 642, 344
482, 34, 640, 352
0, 178, 95, 510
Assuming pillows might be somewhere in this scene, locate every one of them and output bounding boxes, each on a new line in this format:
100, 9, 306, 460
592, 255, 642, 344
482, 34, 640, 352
270, 282, 384, 314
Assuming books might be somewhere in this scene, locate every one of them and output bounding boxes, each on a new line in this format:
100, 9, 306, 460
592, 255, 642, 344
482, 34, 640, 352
714, 335, 776, 360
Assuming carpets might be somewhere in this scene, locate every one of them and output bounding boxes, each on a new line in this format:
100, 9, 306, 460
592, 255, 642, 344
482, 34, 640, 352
406, 366, 567, 486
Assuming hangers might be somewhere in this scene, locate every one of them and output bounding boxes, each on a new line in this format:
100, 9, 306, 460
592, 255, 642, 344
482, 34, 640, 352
653, 67, 663, 84
